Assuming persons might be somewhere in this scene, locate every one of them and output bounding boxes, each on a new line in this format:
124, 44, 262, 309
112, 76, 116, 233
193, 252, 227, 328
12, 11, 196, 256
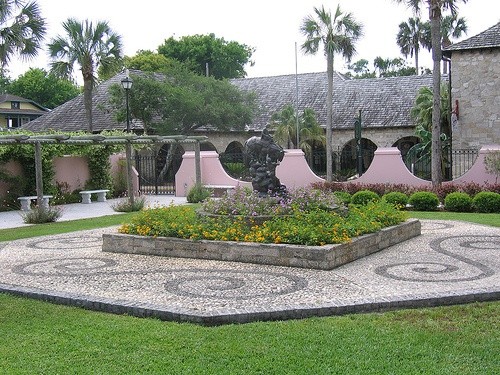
247, 129, 284, 192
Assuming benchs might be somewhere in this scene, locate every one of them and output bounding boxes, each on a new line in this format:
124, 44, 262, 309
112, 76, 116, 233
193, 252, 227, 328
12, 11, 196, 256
78, 189, 110, 204
17, 194, 54, 211
200, 185, 236, 197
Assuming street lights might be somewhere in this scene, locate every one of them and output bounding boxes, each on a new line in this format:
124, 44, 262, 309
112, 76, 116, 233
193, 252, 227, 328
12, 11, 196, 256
121, 76, 133, 134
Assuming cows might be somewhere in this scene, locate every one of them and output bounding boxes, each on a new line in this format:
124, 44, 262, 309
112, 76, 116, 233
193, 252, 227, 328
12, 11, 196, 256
242, 136, 286, 163
